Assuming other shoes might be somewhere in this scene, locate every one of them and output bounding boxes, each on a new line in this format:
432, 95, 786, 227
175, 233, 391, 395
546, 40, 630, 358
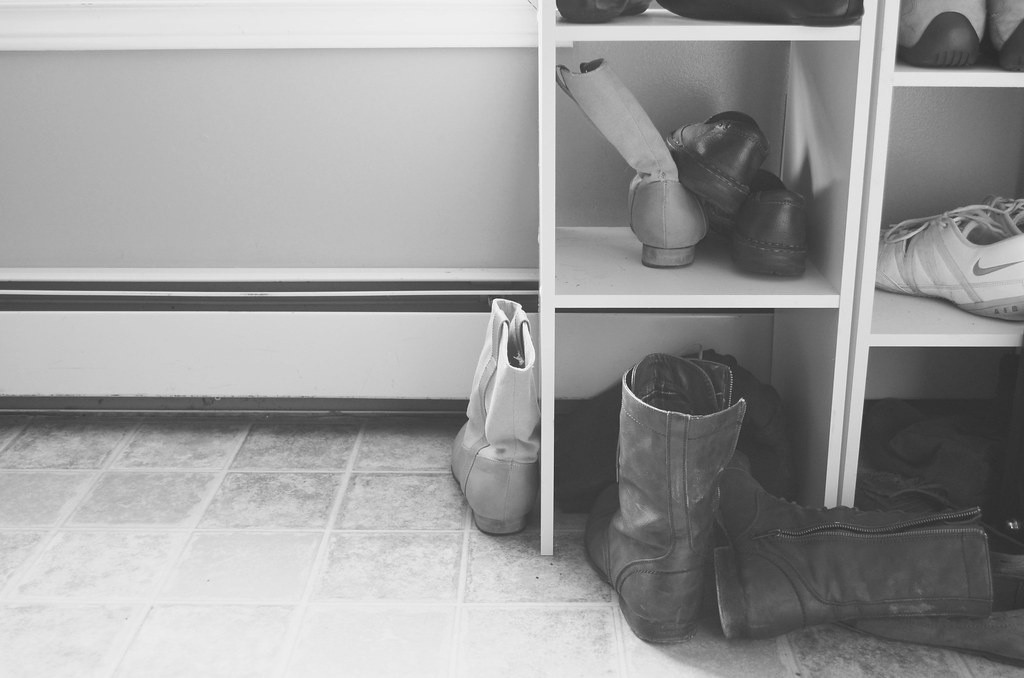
834, 466, 1024, 667
898, 0, 1024, 73
555, 0, 864, 27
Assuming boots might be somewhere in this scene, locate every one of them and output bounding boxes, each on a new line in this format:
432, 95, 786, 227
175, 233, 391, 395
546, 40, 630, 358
712, 447, 993, 641
555, 58, 710, 270
583, 351, 746, 645
448, 298, 542, 536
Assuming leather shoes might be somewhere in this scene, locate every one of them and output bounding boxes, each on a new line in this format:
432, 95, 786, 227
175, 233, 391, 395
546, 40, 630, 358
664, 110, 769, 218
704, 168, 810, 278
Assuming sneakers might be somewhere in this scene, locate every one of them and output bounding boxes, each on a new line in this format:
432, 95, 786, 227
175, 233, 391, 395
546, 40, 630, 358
875, 193, 1024, 321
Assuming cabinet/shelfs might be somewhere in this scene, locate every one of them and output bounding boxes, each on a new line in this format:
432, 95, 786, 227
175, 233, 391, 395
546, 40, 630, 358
535, 0, 1024, 555
